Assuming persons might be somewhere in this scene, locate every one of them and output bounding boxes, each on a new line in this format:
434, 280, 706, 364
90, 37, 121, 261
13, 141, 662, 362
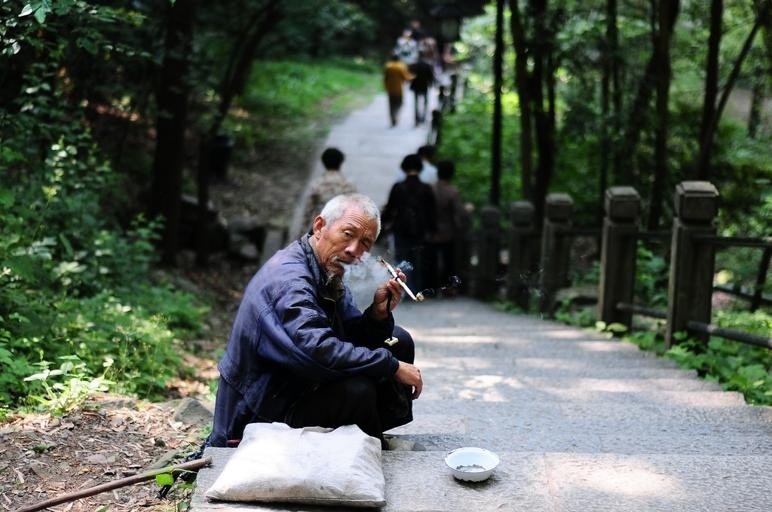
381, 146, 472, 303
384, 21, 439, 125
210, 193, 426, 451
298, 148, 360, 240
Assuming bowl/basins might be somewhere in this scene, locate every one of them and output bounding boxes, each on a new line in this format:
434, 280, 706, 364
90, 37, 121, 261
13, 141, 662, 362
445, 447, 499, 483
